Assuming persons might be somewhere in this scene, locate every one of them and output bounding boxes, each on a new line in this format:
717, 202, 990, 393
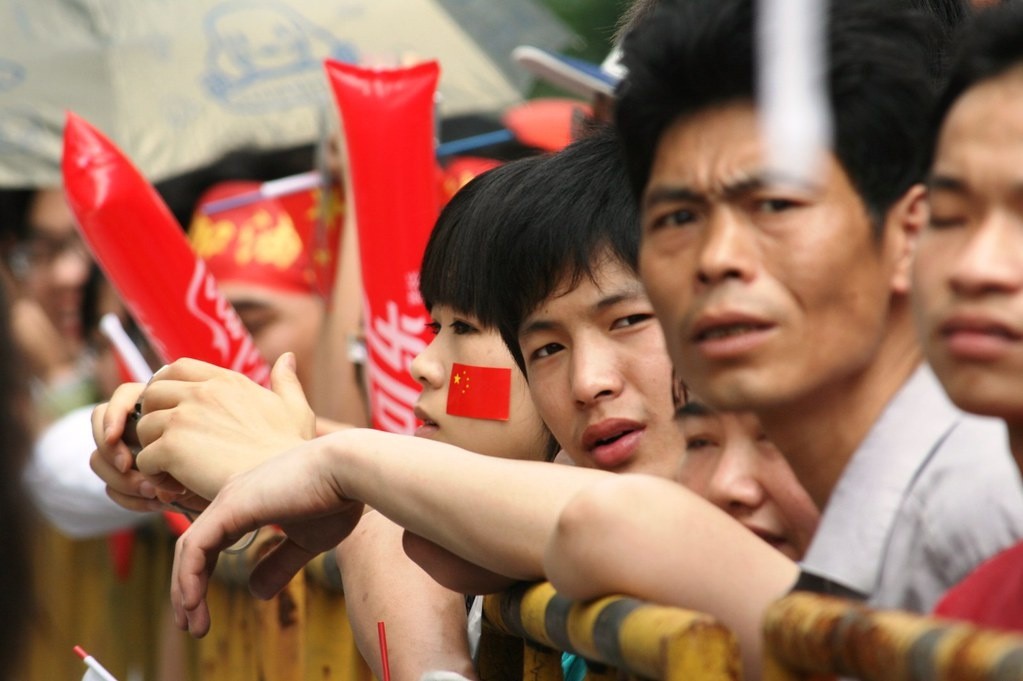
6, 2, 1023, 681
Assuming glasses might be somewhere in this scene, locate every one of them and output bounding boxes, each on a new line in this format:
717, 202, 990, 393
9, 226, 82, 284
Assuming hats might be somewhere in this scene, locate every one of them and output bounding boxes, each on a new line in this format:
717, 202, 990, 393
512, 36, 630, 100
